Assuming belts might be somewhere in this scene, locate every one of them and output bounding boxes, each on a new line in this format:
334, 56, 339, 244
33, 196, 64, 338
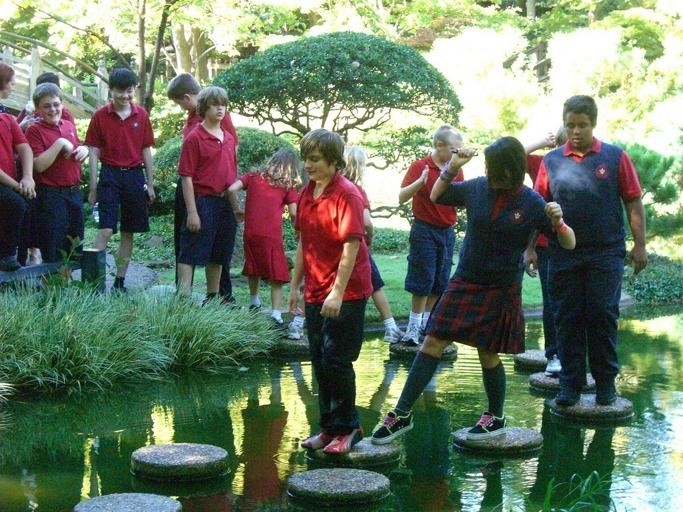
111, 166, 142, 170
39, 185, 79, 193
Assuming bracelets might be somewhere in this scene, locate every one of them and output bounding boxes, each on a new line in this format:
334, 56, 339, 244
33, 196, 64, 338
553, 219, 566, 233
440, 170, 458, 182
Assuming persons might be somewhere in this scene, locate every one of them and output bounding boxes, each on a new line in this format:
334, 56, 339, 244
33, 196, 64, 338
399, 128, 464, 345
0, 60, 87, 270
371, 137, 579, 445
524, 132, 583, 377
177, 86, 236, 304
168, 74, 239, 305
525, 97, 648, 406
286, 128, 374, 453
229, 149, 296, 325
284, 148, 404, 344
85, 67, 156, 302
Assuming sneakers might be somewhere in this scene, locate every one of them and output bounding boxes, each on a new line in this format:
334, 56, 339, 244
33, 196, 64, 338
0, 256, 21, 271
384, 325, 426, 346
287, 323, 304, 340
596, 383, 616, 404
545, 358, 562, 376
301, 412, 414, 454
467, 412, 505, 440
556, 387, 580, 405
